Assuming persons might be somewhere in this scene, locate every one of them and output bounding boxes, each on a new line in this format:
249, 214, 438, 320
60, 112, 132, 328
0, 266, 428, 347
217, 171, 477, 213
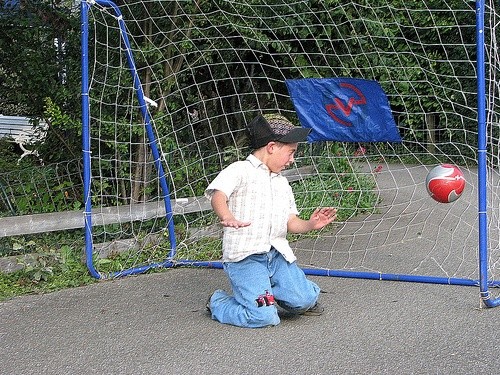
204, 114, 338, 328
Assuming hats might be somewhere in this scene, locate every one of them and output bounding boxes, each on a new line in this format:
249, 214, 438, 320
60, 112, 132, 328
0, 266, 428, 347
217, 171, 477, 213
245, 113, 312, 148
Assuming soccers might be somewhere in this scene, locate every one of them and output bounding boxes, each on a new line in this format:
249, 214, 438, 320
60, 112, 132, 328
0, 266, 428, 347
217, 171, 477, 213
424, 163, 466, 203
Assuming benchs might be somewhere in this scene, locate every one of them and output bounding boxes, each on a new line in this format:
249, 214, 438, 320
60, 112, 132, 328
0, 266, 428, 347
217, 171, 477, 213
0, 115, 54, 165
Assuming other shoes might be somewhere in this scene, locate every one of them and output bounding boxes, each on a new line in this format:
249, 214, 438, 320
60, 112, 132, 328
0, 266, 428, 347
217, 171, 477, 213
203, 289, 225, 315
302, 301, 324, 316
